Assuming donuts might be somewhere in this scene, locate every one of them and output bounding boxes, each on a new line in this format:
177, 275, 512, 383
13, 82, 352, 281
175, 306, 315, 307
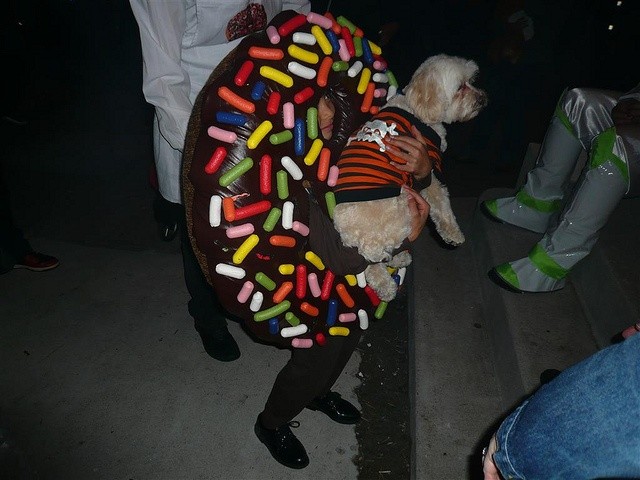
181, 10, 410, 356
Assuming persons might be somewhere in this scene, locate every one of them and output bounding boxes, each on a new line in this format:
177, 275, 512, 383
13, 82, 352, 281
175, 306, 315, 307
254, 92, 433, 470
468, 0, 587, 171
481, 83, 640, 293
128, 0, 312, 362
0, 169, 61, 275
481, 331, 639, 476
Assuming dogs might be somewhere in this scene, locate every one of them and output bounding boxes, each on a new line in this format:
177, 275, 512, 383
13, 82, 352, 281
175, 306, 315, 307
332, 54, 488, 303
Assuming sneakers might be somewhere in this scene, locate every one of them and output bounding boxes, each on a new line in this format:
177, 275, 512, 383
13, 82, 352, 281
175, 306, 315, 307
13, 239, 60, 271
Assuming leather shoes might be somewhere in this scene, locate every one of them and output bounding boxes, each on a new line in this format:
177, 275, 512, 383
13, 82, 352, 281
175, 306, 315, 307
187, 297, 242, 362
254, 419, 310, 469
305, 390, 361, 424
